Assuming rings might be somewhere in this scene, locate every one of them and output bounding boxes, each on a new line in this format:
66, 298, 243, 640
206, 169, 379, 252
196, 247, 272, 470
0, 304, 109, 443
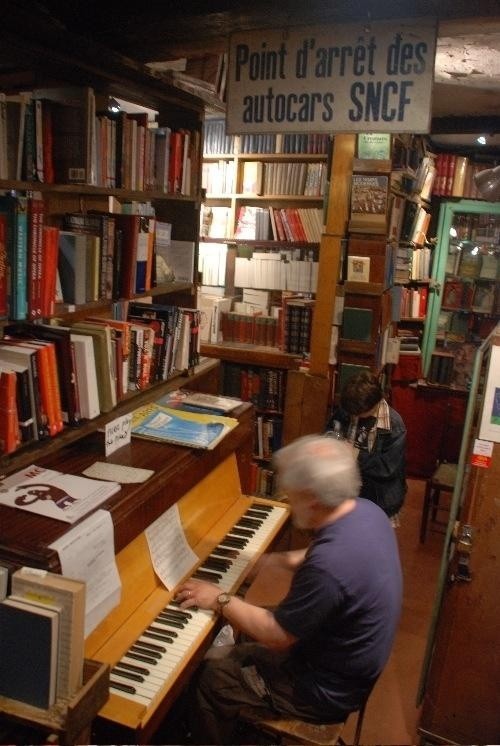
189, 592, 191, 595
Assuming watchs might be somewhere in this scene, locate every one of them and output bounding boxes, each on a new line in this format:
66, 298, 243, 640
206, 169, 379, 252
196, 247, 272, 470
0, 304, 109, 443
216, 592, 231, 614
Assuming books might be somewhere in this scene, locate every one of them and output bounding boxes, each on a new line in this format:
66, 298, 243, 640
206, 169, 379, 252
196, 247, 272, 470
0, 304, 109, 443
0, 94, 202, 458
198, 118, 330, 498
336, 132, 500, 391
0, 566, 83, 709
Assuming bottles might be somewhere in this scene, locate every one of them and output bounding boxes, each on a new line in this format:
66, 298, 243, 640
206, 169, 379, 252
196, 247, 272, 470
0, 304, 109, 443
457, 525, 474, 556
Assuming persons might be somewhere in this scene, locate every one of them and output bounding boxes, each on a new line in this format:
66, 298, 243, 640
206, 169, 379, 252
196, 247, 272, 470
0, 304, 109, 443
174, 433, 403, 723
326, 368, 408, 517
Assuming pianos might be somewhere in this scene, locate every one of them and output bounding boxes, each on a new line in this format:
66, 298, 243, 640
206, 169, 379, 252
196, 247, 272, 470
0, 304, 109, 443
0, 391, 293, 745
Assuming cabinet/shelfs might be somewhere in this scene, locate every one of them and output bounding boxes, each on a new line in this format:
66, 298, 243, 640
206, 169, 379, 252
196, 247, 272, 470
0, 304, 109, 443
338, 142, 432, 380
1, 9, 220, 474
423, 201, 499, 387
197, 114, 358, 499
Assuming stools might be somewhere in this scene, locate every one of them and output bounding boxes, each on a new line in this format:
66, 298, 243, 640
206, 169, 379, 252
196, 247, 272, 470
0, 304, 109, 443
419, 464, 457, 544
238, 707, 351, 745
